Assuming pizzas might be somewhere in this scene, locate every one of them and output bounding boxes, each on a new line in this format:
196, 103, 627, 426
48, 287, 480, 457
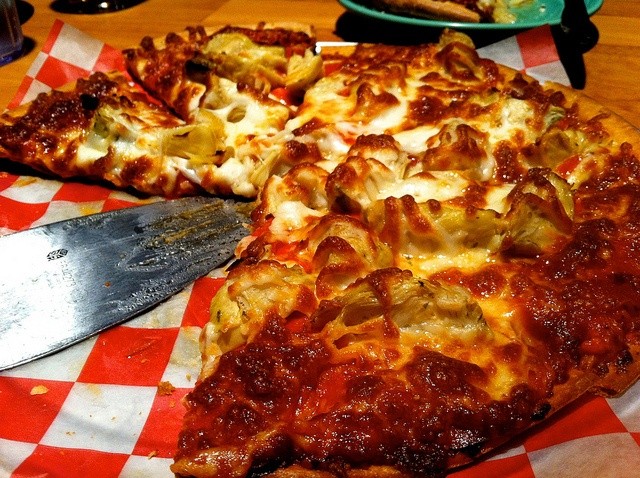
2, 23, 640, 476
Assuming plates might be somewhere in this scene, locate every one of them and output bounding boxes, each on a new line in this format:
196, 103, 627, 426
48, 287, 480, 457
339, 0, 607, 31
